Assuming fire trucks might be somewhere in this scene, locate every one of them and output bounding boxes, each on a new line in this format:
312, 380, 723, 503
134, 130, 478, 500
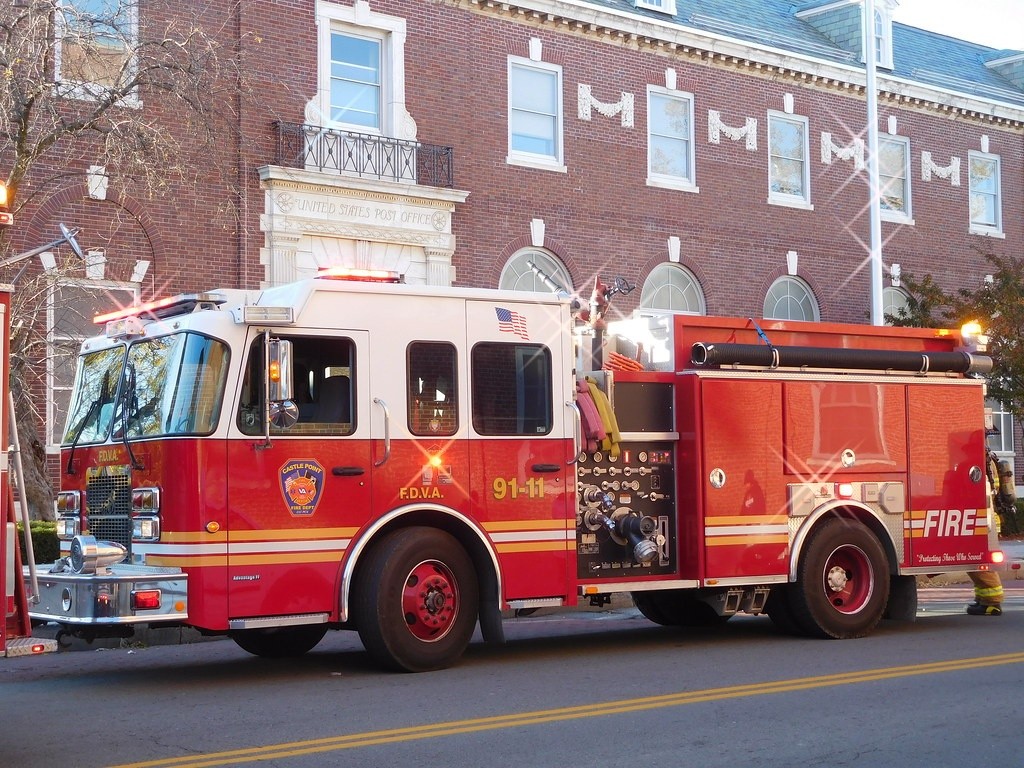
0, 263, 1006, 672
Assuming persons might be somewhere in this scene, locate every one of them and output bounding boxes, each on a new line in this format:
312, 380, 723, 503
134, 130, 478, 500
966, 451, 1004, 615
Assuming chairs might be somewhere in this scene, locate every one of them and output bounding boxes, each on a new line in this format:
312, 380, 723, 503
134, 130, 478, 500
310, 375, 350, 424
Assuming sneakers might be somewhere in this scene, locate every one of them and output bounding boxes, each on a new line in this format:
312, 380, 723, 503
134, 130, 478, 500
967, 604, 1002, 616
